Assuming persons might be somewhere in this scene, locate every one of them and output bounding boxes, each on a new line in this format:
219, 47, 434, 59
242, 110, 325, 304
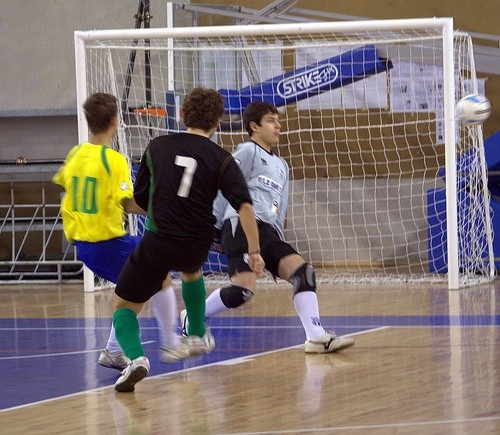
112, 88, 265, 393
181, 103, 354, 354
53, 92, 190, 371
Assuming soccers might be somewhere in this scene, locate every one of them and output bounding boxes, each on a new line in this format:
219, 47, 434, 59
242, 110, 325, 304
457, 94, 491, 126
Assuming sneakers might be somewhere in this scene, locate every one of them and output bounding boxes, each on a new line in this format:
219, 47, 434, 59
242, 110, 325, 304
115, 356, 150, 392
180, 308, 188, 339
98, 348, 130, 371
159, 341, 191, 363
188, 330, 216, 357
304, 334, 355, 354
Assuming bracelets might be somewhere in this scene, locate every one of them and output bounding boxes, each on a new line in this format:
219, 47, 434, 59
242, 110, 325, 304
249, 251, 260, 255
